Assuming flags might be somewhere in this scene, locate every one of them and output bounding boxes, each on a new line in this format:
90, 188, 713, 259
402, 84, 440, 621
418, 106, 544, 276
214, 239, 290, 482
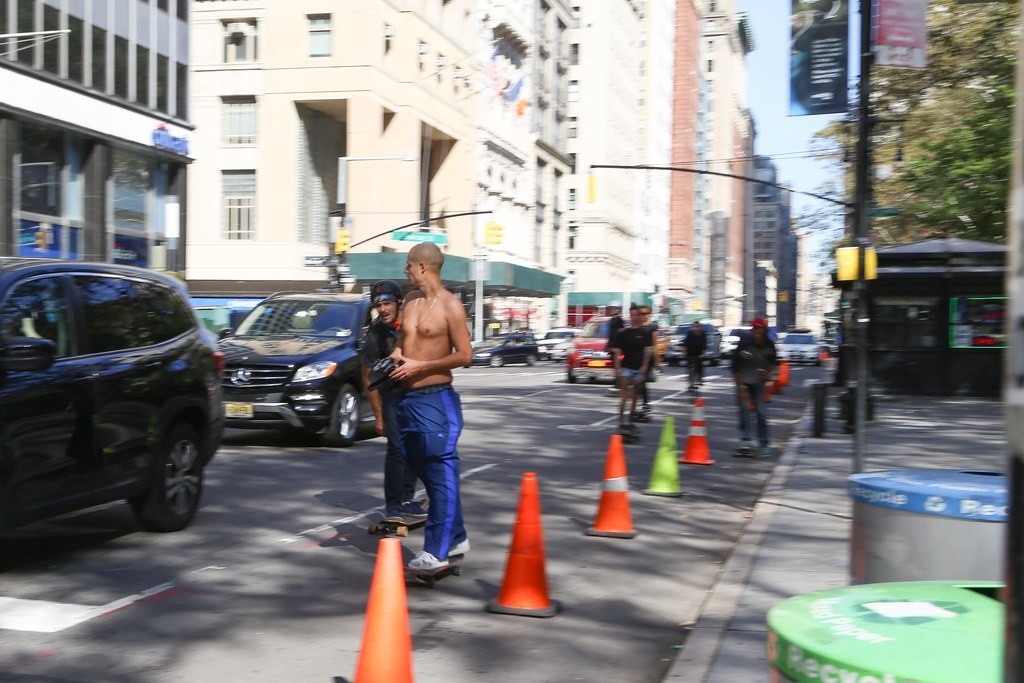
482, 44, 528, 115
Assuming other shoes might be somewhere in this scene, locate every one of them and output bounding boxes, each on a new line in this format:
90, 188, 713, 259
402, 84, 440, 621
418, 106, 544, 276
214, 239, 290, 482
759, 444, 771, 459
741, 440, 752, 451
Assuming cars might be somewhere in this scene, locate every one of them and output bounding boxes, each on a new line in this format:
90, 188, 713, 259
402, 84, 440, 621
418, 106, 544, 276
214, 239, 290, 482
465, 333, 540, 368
665, 324, 722, 367
720, 326, 754, 360
0, 256, 224, 533
656, 329, 672, 363
566, 315, 631, 384
782, 333, 820, 366
536, 327, 583, 362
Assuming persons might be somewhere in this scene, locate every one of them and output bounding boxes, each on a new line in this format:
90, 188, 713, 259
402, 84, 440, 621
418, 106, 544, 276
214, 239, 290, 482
360, 281, 428, 521
824, 327, 842, 345
682, 320, 708, 388
387, 241, 472, 570
333, 311, 354, 331
733, 317, 777, 456
605, 306, 659, 431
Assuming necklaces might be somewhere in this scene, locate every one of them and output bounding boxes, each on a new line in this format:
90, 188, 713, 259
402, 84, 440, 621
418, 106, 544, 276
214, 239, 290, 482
413, 287, 443, 327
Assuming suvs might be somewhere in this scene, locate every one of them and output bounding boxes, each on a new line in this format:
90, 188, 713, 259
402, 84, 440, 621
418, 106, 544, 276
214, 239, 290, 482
218, 291, 378, 449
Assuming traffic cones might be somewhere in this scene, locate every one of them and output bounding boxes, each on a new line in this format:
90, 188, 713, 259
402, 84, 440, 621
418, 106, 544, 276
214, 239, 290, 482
641, 415, 684, 497
764, 362, 790, 402
486, 473, 562, 618
354, 538, 415, 682
585, 434, 639, 538
678, 399, 717, 463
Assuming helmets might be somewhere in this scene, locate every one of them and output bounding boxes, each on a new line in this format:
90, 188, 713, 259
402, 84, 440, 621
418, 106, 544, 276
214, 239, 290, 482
372, 280, 401, 304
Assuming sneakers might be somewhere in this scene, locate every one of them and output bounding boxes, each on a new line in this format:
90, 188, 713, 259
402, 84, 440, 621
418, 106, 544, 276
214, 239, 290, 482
399, 501, 429, 517
408, 550, 449, 571
385, 504, 403, 521
448, 538, 470, 557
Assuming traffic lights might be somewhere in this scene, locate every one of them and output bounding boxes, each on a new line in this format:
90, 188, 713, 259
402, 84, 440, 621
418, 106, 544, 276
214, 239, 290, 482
485, 223, 502, 246
335, 228, 350, 255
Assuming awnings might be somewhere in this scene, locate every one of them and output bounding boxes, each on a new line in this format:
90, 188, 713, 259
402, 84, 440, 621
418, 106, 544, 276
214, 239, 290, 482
189, 296, 295, 314
